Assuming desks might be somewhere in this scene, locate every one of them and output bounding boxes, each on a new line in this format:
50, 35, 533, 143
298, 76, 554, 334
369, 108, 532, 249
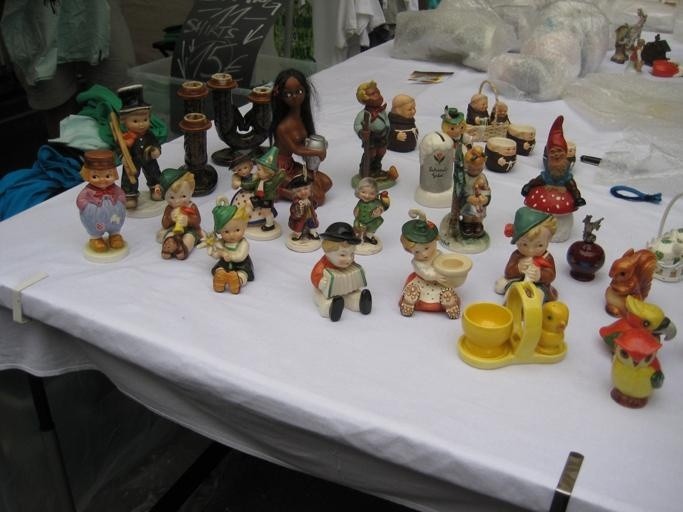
0, 0, 683, 512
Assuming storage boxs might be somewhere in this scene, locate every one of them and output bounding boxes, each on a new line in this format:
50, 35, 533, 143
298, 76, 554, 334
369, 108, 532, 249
124, 47, 325, 133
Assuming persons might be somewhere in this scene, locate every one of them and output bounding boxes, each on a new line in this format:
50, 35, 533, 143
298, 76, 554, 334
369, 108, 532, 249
76, 69, 678, 410
76, 69, 586, 322
